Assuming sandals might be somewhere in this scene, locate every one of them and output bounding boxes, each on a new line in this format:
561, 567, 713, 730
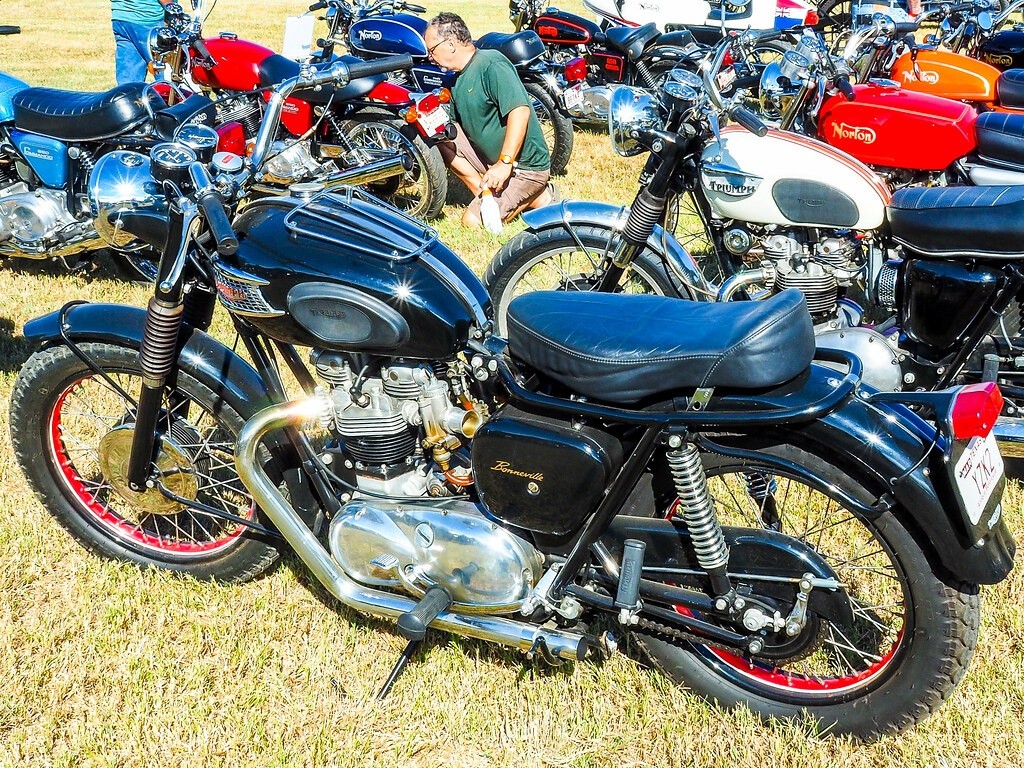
541, 180, 564, 209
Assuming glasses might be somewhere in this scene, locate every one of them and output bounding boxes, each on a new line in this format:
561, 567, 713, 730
426, 38, 451, 55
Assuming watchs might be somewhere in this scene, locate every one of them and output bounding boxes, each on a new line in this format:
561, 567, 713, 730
499, 154, 518, 167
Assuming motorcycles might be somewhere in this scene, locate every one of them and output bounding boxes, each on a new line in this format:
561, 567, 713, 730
1, 24, 218, 290
482, 1, 1024, 463
145, 0, 458, 226
308, 1, 1024, 444
8, 52, 1018, 749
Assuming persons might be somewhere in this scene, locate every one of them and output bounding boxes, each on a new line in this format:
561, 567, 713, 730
422, 10, 560, 230
108, 0, 183, 85
